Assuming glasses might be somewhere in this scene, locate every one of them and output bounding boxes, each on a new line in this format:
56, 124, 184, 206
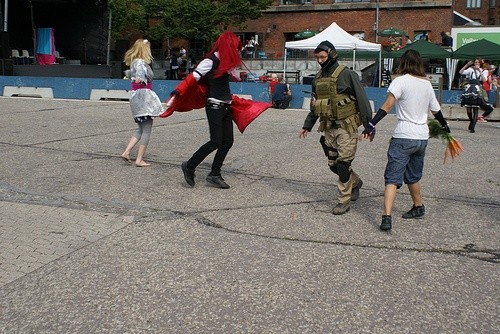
315, 56, 328, 60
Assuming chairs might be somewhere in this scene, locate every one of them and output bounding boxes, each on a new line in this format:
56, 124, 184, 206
55, 51, 65, 64
22, 49, 35, 64
12, 49, 25, 65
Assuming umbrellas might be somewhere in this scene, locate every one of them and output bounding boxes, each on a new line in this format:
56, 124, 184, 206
376, 28, 407, 37
294, 29, 322, 38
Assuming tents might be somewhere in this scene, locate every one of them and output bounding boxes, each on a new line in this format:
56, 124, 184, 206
282, 21, 382, 88
451, 39, 500, 91
381, 39, 453, 90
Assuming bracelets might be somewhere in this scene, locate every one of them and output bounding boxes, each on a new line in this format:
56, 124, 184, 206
467, 63, 470, 67
368, 121, 375, 128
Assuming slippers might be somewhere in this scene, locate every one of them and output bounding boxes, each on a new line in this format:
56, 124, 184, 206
136, 160, 151, 167
121, 156, 131, 164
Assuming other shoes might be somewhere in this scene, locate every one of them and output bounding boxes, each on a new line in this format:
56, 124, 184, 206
273, 104, 286, 110
477, 115, 488, 123
350, 178, 364, 201
468, 121, 476, 133
332, 202, 353, 216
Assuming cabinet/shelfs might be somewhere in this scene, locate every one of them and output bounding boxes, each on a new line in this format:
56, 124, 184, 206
382, 69, 391, 88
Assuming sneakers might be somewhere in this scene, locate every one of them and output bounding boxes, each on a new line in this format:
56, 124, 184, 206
180, 161, 196, 186
380, 214, 392, 230
206, 173, 230, 189
401, 204, 427, 219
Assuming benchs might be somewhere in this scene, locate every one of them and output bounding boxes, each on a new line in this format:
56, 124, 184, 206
302, 96, 375, 113
89, 89, 131, 100
233, 93, 253, 101
2, 85, 54, 98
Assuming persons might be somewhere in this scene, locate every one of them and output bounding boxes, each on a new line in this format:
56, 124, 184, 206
169, 45, 199, 80
236, 35, 260, 59
382, 35, 402, 48
158, 30, 275, 190
439, 31, 453, 47
120, 38, 165, 167
458, 57, 500, 134
358, 49, 451, 230
298, 40, 375, 215
269, 74, 293, 110
405, 36, 412, 45
